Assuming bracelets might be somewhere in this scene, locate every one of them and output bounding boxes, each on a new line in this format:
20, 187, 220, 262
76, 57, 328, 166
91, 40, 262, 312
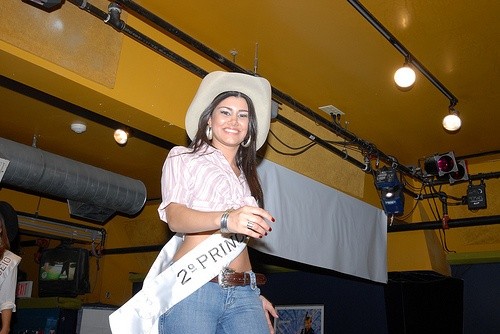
221, 207, 234, 233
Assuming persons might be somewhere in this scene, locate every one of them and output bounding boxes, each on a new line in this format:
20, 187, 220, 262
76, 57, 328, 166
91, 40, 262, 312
61, 255, 70, 280
300, 317, 315, 334
0, 214, 21, 334
158, 71, 278, 334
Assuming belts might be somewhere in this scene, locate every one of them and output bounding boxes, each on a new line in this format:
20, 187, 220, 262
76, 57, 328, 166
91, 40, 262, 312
210, 270, 266, 287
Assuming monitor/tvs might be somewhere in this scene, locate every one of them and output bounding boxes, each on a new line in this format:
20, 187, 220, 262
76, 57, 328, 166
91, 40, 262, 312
38, 247, 82, 297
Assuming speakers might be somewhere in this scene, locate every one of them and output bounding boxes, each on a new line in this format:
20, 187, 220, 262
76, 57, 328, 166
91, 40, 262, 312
375, 170, 405, 214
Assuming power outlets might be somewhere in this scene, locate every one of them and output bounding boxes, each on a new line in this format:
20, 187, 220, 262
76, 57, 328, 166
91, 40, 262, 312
319, 105, 345, 117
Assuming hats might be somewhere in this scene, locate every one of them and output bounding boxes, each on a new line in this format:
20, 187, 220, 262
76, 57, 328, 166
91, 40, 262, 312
184, 71, 272, 153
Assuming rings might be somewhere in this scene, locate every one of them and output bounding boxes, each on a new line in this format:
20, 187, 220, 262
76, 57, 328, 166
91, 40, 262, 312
247, 221, 254, 229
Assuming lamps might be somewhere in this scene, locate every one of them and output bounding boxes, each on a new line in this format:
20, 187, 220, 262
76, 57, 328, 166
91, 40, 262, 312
442, 100, 462, 131
393, 55, 417, 88
373, 162, 405, 217
